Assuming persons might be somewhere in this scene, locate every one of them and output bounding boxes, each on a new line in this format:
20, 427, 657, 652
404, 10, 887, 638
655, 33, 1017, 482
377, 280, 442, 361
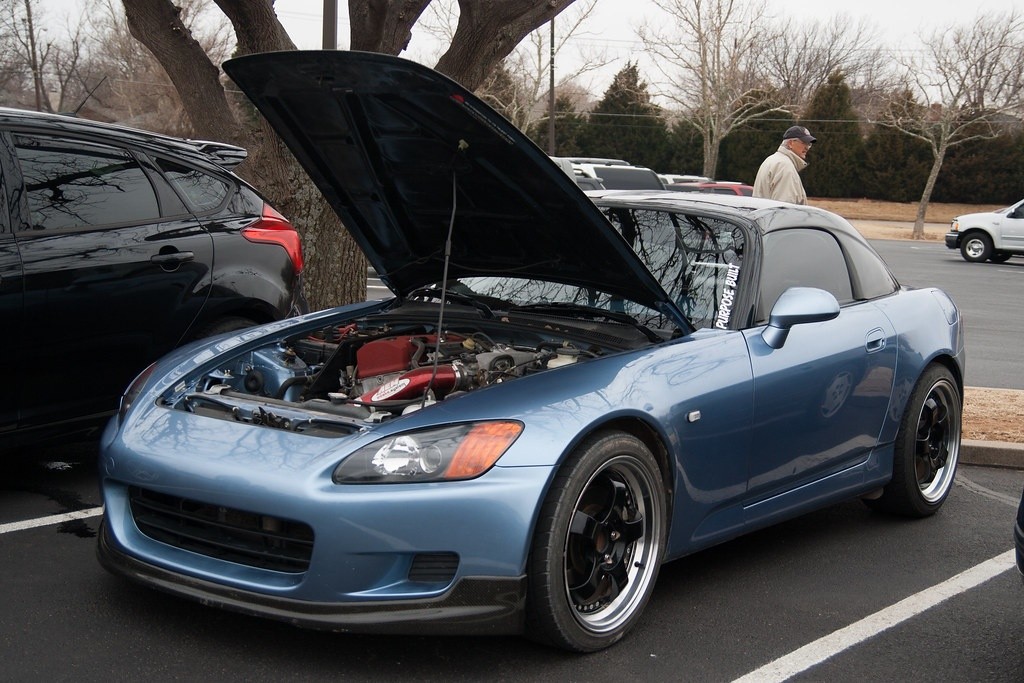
752, 124, 817, 206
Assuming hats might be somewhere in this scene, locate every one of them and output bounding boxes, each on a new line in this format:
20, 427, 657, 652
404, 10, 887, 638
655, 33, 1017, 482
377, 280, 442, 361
783, 126, 816, 144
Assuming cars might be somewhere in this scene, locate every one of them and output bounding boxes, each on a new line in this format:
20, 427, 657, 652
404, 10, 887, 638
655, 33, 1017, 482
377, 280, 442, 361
92, 50, 968, 652
547, 155, 666, 191
0, 105, 310, 490
657, 172, 713, 183
945, 199, 1023, 263
664, 182, 755, 198
